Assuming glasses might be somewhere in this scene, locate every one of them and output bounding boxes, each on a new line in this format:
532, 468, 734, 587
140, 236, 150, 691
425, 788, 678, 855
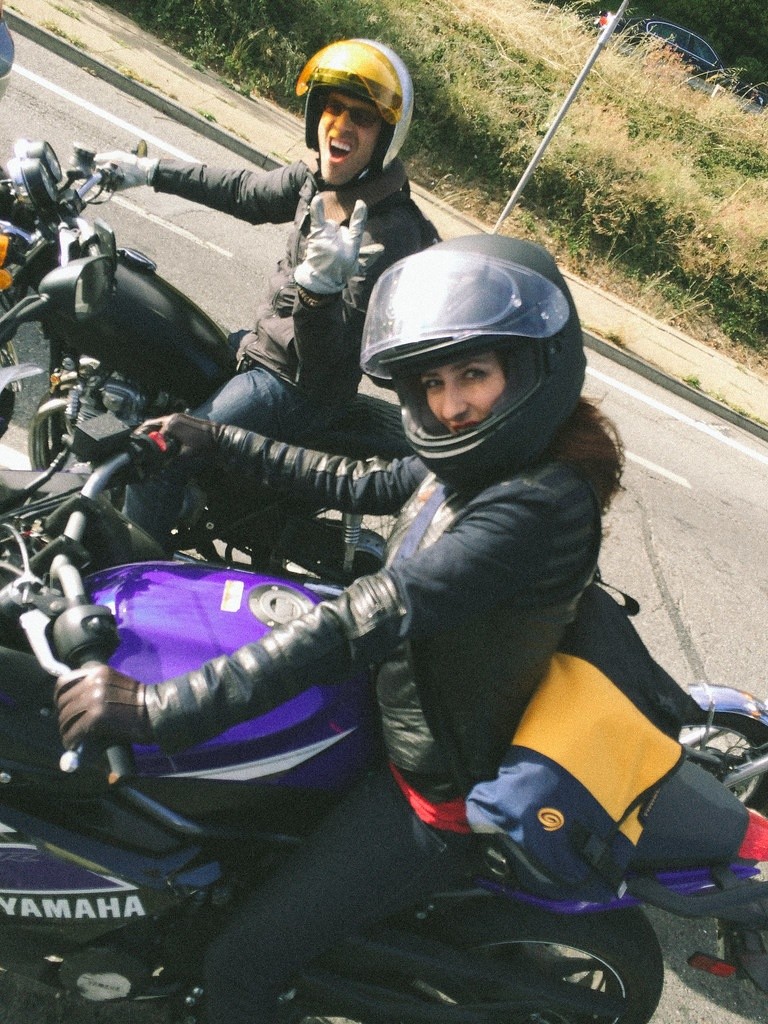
318, 95, 383, 129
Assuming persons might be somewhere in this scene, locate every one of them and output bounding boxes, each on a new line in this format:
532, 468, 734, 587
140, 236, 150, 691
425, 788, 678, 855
53, 235, 625, 1024
98, 39, 443, 546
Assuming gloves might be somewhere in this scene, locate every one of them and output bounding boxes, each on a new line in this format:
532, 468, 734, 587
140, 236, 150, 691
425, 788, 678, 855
93, 150, 159, 191
294, 195, 385, 294
134, 413, 227, 466
53, 666, 154, 754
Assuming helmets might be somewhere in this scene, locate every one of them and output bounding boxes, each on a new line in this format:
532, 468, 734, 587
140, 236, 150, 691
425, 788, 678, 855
305, 39, 414, 170
378, 233, 587, 484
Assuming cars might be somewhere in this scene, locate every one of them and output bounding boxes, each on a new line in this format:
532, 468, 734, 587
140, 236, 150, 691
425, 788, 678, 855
736, 82, 768, 106
591, 11, 739, 92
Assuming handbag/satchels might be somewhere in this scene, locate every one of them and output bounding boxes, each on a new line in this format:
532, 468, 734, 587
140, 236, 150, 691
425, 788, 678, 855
466, 583, 704, 901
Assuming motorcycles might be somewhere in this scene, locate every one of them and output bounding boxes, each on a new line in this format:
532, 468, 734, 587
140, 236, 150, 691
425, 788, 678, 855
0, 136, 765, 1023
